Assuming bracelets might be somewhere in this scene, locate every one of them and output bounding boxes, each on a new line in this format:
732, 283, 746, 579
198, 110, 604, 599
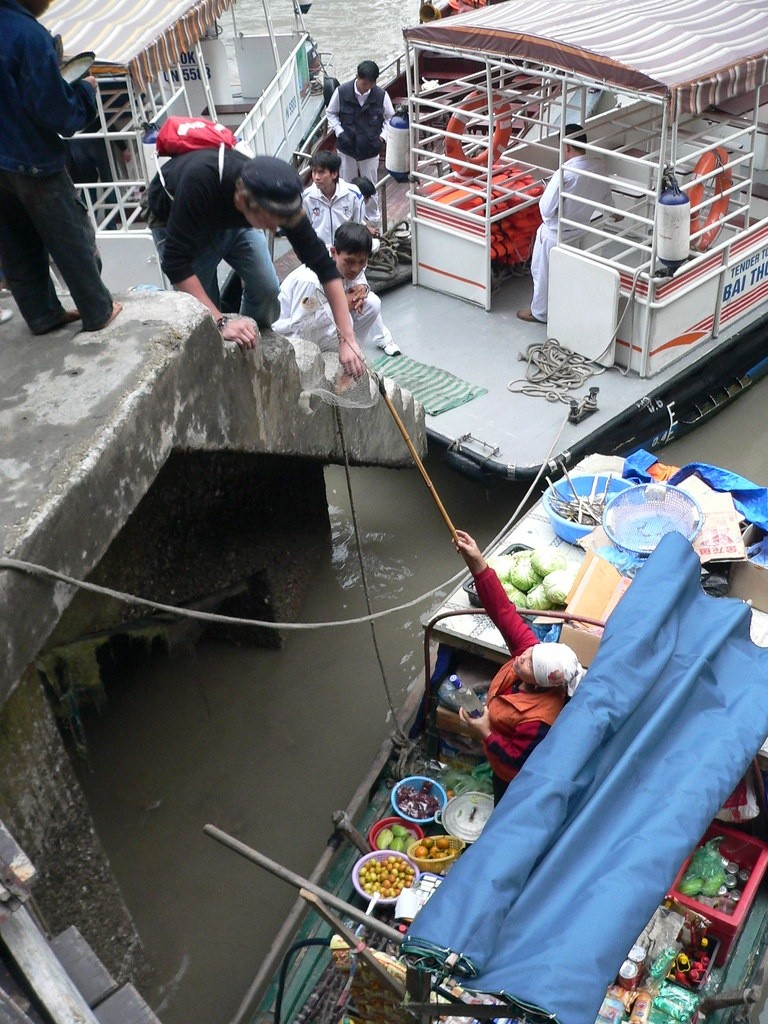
216, 316, 227, 328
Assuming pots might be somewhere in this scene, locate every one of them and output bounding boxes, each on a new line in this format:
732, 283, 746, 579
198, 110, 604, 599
434, 791, 494, 844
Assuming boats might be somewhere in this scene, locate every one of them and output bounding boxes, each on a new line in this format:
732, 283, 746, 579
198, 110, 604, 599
227, 453, 768, 1024
32, 1, 342, 304
329, 1, 768, 487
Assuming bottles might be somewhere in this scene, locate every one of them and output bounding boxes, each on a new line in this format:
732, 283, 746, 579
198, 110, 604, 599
450, 675, 484, 718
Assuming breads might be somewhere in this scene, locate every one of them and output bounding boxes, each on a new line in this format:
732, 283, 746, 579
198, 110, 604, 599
629, 992, 652, 1024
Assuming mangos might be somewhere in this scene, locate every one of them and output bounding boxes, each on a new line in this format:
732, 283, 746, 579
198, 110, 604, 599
377, 824, 417, 854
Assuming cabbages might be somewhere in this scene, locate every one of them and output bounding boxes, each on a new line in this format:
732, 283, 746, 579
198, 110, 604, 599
490, 548, 581, 610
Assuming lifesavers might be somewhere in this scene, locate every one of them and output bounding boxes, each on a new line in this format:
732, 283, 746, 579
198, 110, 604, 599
688, 147, 732, 251
444, 89, 515, 178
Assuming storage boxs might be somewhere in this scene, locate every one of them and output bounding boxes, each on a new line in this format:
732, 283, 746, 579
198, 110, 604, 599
664, 934, 721, 992
462, 543, 535, 606
729, 523, 768, 613
670, 822, 768, 965
531, 549, 632, 668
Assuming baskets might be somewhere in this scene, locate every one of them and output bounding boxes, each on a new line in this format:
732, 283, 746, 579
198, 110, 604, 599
463, 543, 551, 621
352, 775, 467, 904
602, 482, 708, 553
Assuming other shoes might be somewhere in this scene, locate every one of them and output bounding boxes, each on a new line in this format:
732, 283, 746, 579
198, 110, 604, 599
517, 309, 540, 322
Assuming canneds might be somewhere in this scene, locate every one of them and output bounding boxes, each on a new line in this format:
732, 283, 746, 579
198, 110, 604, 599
716, 862, 751, 901
617, 946, 646, 990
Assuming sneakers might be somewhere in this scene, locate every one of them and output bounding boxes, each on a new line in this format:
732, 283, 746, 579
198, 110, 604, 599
378, 340, 401, 356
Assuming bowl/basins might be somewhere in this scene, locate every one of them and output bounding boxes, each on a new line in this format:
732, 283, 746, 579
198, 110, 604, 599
368, 817, 424, 853
543, 474, 635, 547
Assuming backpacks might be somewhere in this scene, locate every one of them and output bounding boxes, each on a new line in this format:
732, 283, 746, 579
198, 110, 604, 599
152, 116, 237, 202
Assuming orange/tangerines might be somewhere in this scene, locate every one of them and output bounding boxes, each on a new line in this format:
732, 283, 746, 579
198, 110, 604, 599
415, 838, 457, 859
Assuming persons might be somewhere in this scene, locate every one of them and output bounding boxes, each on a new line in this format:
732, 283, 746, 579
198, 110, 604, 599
326, 60, 396, 227
139, 148, 367, 382
517, 124, 624, 323
270, 222, 401, 356
0, 0, 122, 335
301, 150, 380, 271
452, 530, 586, 809
63, 115, 132, 230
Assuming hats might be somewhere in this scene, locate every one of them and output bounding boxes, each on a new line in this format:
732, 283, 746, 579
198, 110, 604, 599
242, 155, 304, 215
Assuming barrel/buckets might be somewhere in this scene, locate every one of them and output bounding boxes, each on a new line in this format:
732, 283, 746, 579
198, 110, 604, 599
666, 938, 711, 986
